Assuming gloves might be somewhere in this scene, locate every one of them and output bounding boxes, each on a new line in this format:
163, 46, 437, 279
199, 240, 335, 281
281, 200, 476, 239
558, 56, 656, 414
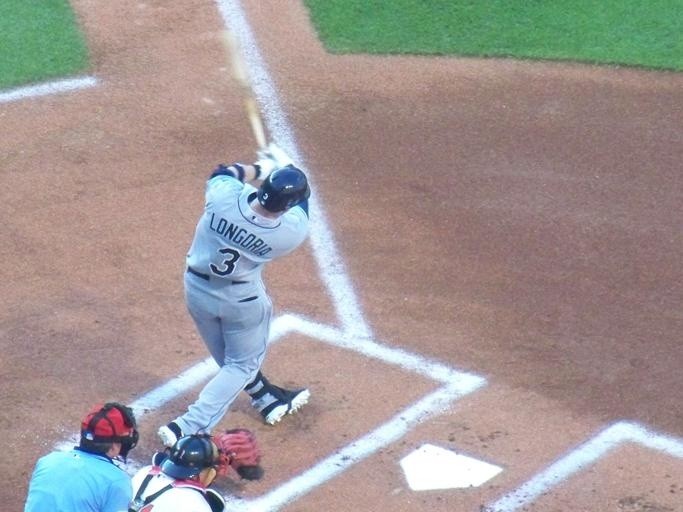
263, 142, 297, 167
251, 150, 275, 181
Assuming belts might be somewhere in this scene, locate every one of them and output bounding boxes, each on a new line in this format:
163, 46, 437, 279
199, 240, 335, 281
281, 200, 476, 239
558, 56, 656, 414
185, 265, 249, 286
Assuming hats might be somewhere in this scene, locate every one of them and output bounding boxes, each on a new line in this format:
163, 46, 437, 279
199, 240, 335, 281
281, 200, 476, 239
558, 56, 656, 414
78, 403, 131, 438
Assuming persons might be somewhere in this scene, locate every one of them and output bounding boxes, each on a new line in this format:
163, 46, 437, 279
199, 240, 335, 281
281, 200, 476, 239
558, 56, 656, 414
156, 143, 312, 446
21, 400, 133, 512
133, 426, 264, 512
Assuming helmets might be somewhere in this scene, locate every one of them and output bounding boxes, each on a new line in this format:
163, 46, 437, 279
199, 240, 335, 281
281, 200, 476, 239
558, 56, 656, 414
254, 165, 311, 214
158, 434, 220, 481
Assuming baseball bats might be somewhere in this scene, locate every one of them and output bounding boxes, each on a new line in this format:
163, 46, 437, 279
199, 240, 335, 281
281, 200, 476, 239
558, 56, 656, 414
216, 28, 266, 149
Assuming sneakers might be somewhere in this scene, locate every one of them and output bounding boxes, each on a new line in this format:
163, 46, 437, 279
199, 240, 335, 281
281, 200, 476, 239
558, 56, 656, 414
155, 420, 183, 449
252, 382, 310, 427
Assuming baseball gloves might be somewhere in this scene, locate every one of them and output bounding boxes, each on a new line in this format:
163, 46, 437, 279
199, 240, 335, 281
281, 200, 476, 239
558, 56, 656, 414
211, 427, 264, 480
